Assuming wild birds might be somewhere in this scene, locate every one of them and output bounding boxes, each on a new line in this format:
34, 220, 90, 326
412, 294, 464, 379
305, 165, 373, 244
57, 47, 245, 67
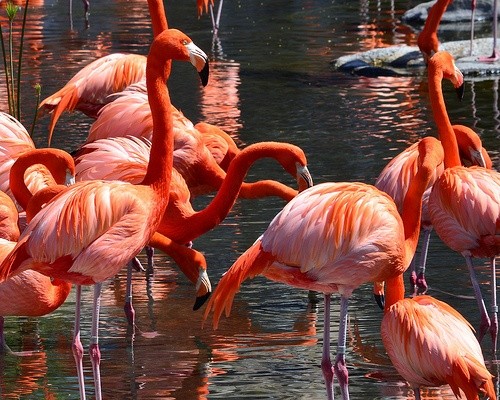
0, 0, 500, 400
